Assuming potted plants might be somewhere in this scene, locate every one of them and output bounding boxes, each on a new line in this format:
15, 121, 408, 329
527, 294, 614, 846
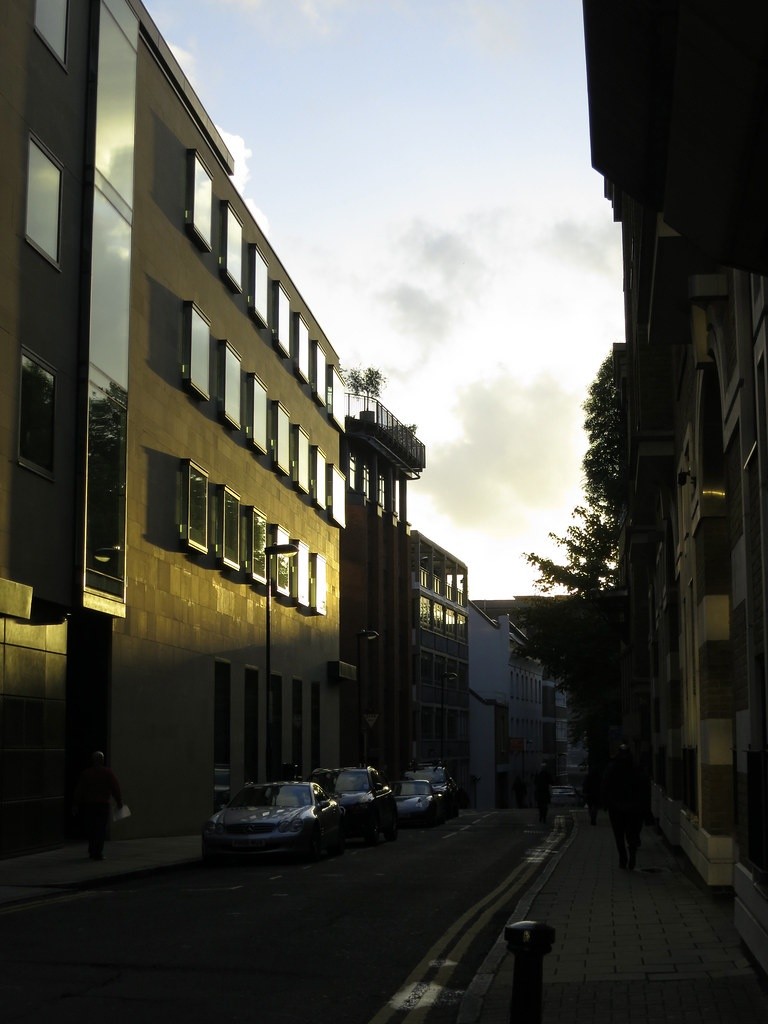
347, 367, 384, 423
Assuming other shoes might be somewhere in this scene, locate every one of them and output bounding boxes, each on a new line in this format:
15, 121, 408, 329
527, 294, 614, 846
620, 854, 626, 870
629, 851, 637, 871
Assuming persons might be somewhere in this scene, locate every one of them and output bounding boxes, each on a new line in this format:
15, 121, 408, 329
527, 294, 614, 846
511, 776, 528, 809
69, 751, 123, 861
585, 769, 602, 825
602, 744, 652, 869
532, 764, 553, 823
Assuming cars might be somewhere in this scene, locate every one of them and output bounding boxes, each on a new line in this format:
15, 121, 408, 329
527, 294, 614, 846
391, 780, 441, 829
201, 780, 346, 870
550, 786, 582, 808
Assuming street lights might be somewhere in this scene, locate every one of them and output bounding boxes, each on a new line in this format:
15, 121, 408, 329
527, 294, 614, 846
265, 542, 299, 784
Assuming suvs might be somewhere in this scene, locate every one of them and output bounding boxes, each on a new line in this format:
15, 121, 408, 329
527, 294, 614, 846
404, 759, 460, 820
306, 765, 399, 847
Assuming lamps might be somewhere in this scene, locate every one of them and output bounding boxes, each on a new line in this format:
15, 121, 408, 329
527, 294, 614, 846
677, 470, 690, 485
